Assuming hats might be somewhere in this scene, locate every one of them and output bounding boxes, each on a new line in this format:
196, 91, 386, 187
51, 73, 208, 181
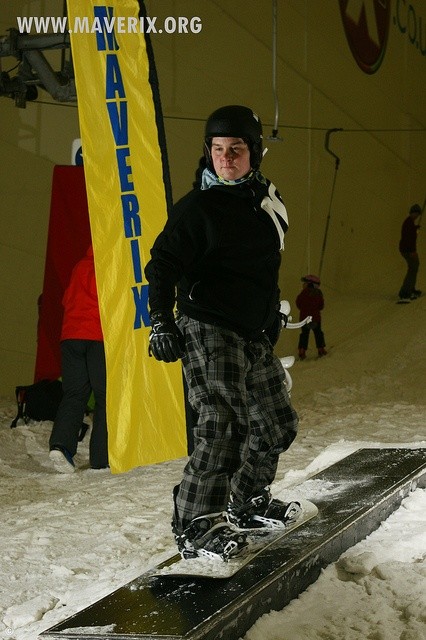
409, 204, 421, 214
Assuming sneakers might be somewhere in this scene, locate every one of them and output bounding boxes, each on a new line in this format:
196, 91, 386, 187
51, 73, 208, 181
299, 348, 306, 357
318, 348, 326, 355
173, 484, 249, 563
48, 444, 76, 474
227, 490, 301, 532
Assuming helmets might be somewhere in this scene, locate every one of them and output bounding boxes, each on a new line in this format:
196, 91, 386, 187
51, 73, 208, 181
203, 105, 263, 170
301, 274, 321, 288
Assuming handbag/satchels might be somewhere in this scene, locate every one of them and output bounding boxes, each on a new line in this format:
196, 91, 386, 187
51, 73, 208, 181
11, 379, 92, 442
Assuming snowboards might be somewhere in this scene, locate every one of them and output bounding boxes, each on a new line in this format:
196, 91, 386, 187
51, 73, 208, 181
148, 497, 319, 578
396, 292, 425, 304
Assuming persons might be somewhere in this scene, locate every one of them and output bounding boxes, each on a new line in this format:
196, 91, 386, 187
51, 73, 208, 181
295, 274, 328, 360
143, 105, 304, 563
47, 243, 111, 475
397, 204, 425, 305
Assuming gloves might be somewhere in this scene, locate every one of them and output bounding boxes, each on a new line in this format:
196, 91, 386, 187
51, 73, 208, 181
148, 310, 187, 364
